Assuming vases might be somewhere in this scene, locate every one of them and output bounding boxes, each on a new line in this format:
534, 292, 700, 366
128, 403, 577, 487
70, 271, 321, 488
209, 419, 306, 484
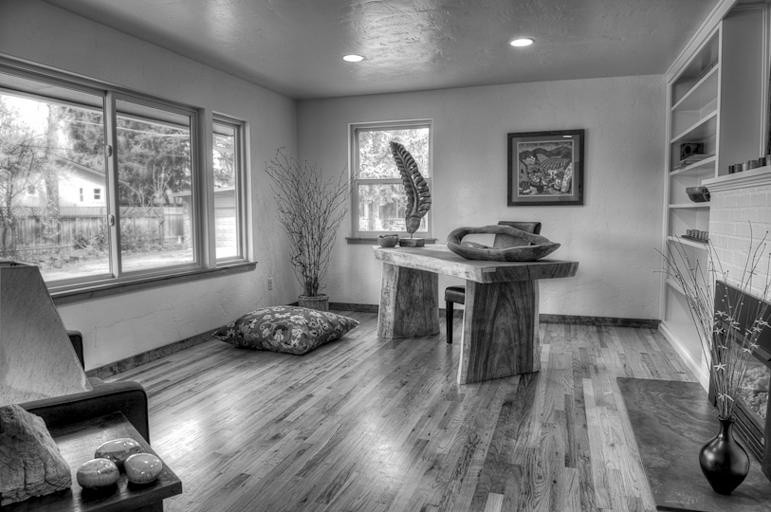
298, 294, 329, 313
700, 412, 755, 497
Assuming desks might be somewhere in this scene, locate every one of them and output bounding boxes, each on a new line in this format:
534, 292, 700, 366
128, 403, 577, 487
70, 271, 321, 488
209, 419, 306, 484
369, 243, 578, 386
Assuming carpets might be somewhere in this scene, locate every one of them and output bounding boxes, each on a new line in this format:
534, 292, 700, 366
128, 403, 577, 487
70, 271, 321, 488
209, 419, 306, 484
614, 367, 770, 512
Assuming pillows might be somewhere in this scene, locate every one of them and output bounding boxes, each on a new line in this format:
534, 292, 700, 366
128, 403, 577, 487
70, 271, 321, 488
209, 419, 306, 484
214, 301, 361, 359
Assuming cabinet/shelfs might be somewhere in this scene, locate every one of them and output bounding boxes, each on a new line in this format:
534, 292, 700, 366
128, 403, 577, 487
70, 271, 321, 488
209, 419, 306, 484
657, 0, 770, 463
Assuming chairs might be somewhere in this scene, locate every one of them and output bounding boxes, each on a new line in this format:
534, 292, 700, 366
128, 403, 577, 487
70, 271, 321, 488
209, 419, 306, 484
444, 220, 541, 344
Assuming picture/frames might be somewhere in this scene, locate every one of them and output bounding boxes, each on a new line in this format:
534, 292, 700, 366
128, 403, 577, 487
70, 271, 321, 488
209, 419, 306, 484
506, 128, 584, 206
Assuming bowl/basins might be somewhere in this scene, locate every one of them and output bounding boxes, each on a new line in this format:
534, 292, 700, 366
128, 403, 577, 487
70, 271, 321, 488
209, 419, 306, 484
685, 186, 710, 202
